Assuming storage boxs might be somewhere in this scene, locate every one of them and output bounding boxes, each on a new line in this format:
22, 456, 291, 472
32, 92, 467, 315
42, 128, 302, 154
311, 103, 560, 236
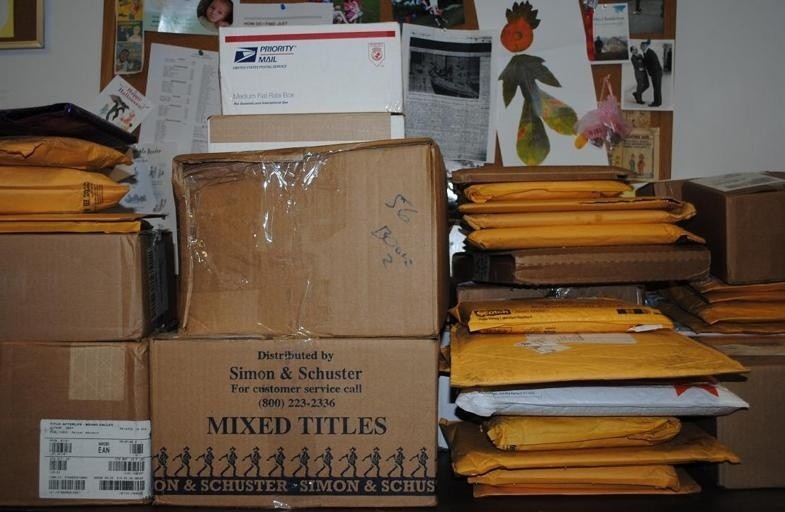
0, 332, 149, 506
217, 22, 403, 114
166, 142, 445, 338
1, 232, 153, 341
137, 333, 440, 509
444, 167, 785, 496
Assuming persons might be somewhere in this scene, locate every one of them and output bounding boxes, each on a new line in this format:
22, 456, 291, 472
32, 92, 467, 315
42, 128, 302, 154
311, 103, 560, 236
630, 41, 663, 107
198, 1, 232, 32
594, 35, 603, 53
